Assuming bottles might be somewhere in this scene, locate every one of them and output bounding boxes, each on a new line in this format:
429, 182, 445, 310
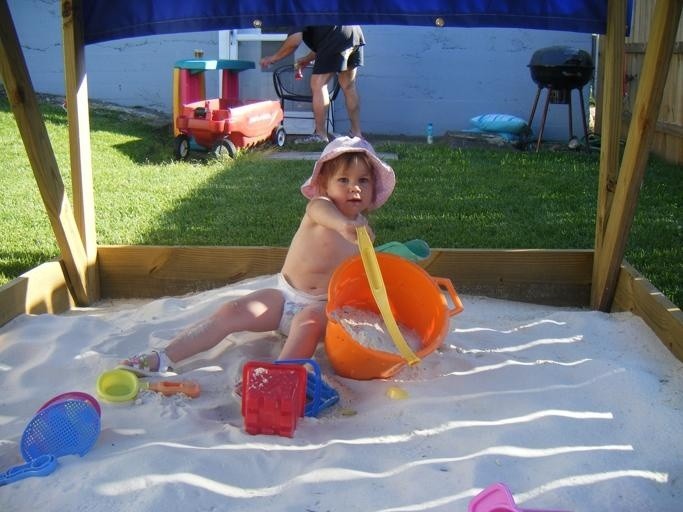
293, 60, 304, 80
427, 123, 433, 144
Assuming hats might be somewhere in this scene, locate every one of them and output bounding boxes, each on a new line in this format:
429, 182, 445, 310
300, 136, 396, 211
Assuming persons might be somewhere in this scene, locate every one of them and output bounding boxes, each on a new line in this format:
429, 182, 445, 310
260, 23, 366, 145
116, 134, 396, 403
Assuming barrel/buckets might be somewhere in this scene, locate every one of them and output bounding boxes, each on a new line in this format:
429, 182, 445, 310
325, 224, 465, 380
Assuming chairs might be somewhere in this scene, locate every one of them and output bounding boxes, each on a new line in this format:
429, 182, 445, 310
273, 63, 340, 136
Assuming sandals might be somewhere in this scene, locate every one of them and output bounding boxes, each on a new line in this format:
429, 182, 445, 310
232, 382, 243, 407
116, 349, 179, 378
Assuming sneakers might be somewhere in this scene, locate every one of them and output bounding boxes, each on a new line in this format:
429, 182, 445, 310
334, 130, 365, 139
295, 131, 328, 145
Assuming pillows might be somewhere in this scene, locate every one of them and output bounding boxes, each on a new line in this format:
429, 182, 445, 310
470, 113, 536, 144
